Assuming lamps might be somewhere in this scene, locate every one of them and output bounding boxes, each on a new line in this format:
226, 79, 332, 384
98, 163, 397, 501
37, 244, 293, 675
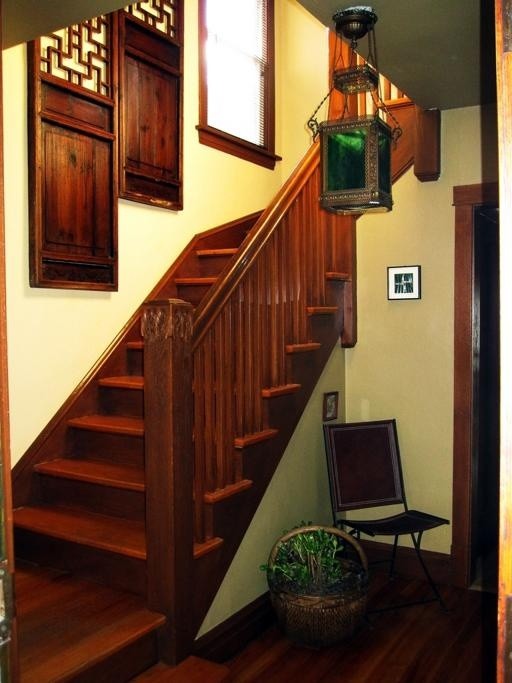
308, 6, 399, 214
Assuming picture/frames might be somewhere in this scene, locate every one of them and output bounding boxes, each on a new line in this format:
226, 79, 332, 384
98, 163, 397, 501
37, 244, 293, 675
386, 266, 421, 301
321, 393, 341, 423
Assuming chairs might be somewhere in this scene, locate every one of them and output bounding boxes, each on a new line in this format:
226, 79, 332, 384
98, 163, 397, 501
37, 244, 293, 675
321, 419, 451, 606
